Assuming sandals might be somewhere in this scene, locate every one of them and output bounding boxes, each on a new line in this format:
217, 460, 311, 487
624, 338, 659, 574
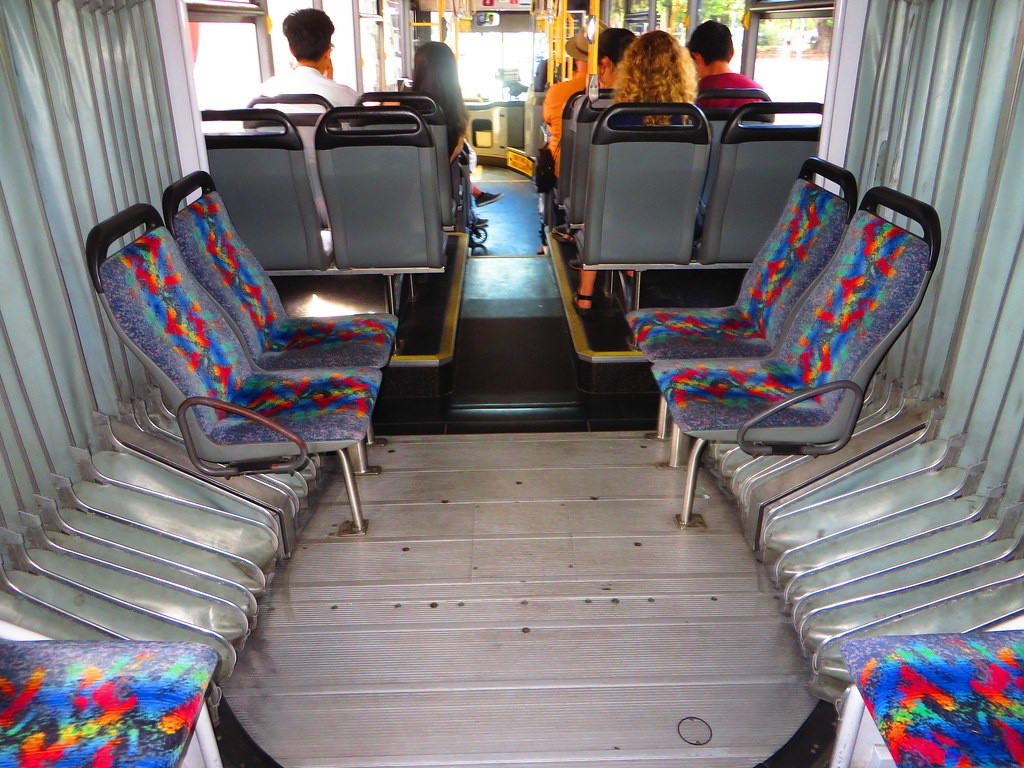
573, 290, 594, 317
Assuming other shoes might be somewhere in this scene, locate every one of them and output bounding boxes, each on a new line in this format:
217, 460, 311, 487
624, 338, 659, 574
536, 244, 546, 255
475, 218, 488, 227
569, 258, 581, 270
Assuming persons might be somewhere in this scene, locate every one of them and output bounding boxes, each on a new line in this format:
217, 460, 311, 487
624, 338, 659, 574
571, 29, 699, 313
534, 43, 577, 92
257, 9, 364, 229
551, 28, 637, 270
535, 28, 606, 255
382, 18, 505, 231
640, 19, 774, 294
479, 14, 499, 26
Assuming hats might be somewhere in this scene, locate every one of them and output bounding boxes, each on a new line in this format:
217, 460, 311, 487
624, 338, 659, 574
565, 18, 608, 62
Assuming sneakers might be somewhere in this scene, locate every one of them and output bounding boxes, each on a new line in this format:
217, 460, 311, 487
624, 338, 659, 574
475, 192, 504, 207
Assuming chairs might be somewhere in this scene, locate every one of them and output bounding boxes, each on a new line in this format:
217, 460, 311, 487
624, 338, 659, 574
0, 82, 1024, 768
